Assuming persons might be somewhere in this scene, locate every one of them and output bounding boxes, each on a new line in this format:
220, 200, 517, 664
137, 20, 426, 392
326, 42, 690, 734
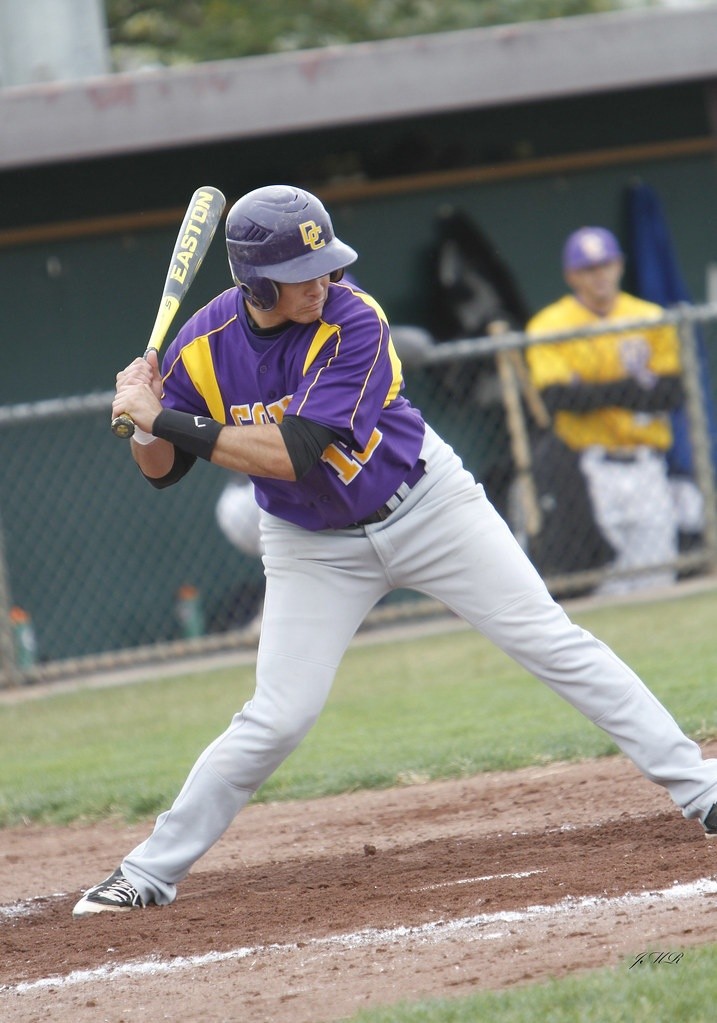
522, 226, 687, 589
70, 185, 717, 915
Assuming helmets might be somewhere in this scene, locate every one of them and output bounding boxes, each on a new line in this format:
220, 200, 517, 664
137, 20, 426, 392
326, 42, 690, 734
225, 186, 360, 312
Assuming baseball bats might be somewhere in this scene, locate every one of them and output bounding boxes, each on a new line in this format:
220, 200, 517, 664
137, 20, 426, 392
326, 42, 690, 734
110, 185, 229, 440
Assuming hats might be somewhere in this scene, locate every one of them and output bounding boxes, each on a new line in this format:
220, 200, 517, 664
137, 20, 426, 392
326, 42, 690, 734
563, 228, 619, 272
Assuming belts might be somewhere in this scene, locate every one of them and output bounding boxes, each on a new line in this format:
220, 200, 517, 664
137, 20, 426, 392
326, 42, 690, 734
350, 456, 427, 528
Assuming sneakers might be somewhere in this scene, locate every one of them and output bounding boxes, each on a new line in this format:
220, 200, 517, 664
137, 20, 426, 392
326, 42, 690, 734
73, 863, 146, 918
702, 803, 717, 840
